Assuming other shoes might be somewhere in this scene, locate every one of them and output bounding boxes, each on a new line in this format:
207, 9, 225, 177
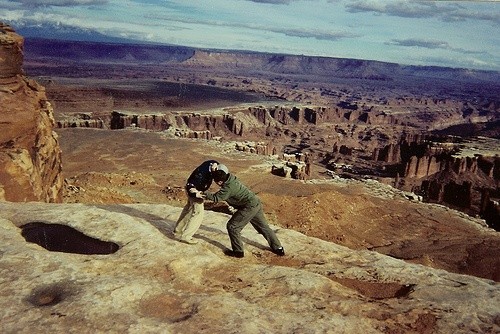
272, 246, 285, 256
171, 231, 198, 244
225, 249, 243, 258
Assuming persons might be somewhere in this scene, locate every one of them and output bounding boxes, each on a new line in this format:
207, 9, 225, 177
189, 164, 285, 256
173, 160, 228, 243
